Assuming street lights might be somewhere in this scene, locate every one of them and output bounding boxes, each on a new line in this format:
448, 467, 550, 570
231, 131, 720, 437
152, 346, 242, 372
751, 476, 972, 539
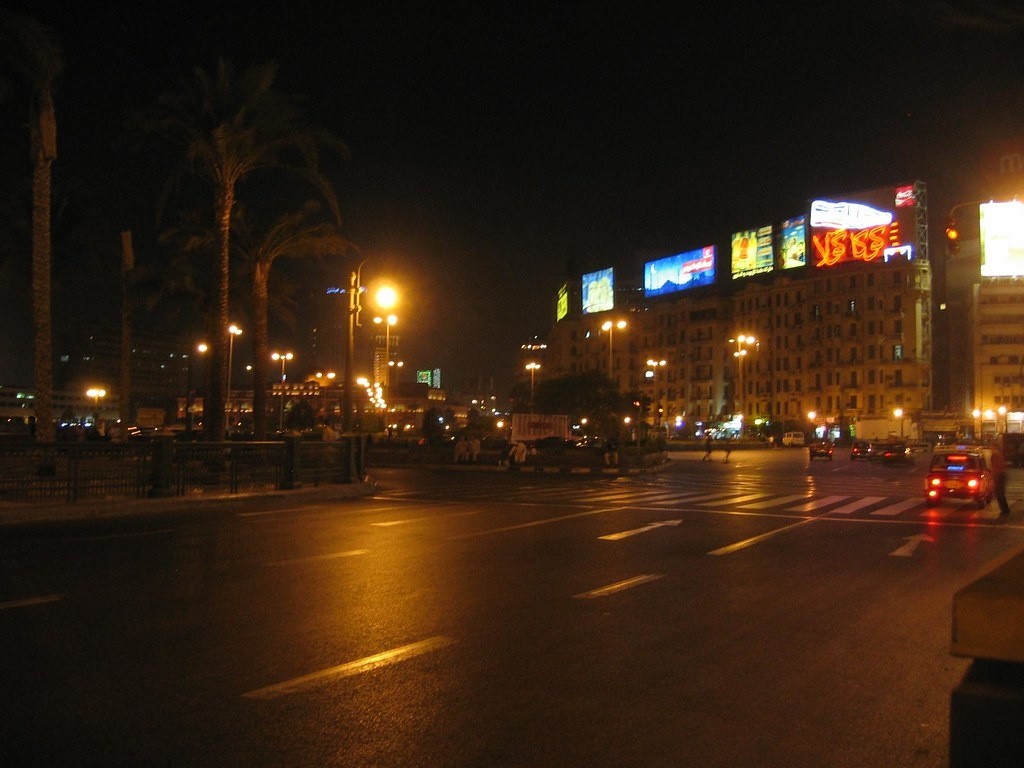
373, 314, 399, 447
525, 362, 542, 414
316, 371, 336, 424
895, 408, 904, 436
645, 359, 667, 427
733, 334, 755, 411
271, 351, 293, 438
86, 388, 106, 423
224, 325, 238, 439
601, 319, 626, 387
185, 343, 208, 439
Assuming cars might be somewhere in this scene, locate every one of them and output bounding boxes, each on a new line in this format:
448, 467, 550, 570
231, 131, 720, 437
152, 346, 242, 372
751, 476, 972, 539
809, 438, 834, 461
303, 424, 328, 440
922, 446, 994, 509
849, 440, 873, 462
880, 445, 914, 467
128, 427, 175, 442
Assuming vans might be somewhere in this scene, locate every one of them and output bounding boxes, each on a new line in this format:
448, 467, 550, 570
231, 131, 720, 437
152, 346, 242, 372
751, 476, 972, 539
782, 431, 805, 448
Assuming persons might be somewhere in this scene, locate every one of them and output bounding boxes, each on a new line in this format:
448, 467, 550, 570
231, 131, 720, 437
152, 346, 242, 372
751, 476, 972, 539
90, 425, 110, 441
498, 440, 526, 465
703, 436, 713, 460
604, 438, 619, 467
787, 236, 804, 262
454, 435, 480, 464
979, 442, 1010, 514
725, 438, 737, 461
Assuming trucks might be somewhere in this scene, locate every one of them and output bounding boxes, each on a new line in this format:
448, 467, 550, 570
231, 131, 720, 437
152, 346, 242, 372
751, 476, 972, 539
508, 412, 591, 448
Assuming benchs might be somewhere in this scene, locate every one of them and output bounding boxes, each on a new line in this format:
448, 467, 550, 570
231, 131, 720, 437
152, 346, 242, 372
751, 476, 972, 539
479, 449, 504, 467
